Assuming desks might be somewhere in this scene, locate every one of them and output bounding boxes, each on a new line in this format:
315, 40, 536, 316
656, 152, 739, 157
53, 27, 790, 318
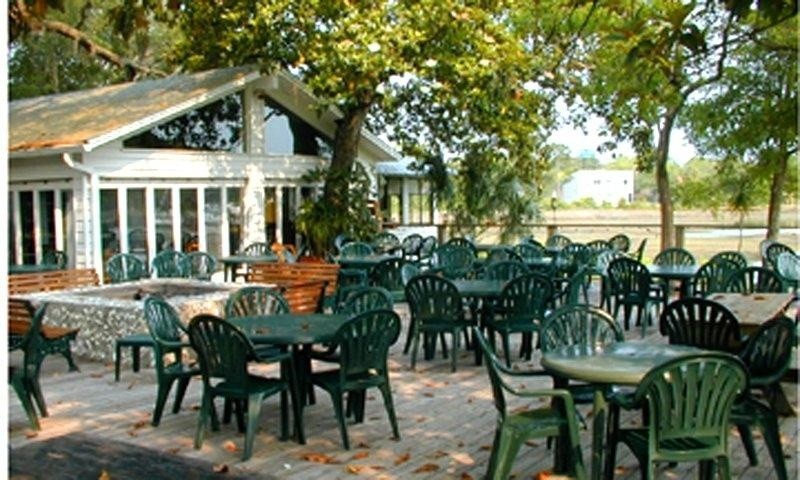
9, 264, 60, 276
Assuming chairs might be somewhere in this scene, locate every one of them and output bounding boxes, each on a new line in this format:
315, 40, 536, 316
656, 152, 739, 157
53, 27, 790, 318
468, 324, 586, 478
8, 301, 50, 430
658, 298, 740, 351
667, 312, 793, 476
146, 296, 238, 433
186, 314, 295, 461
305, 284, 395, 419
603, 356, 741, 478
540, 302, 625, 452
292, 308, 402, 450
43, 250, 68, 270
106, 228, 797, 400
226, 287, 306, 408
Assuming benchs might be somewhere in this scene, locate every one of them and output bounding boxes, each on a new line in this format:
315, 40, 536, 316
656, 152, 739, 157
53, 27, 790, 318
9, 268, 101, 297
8, 298, 82, 372
